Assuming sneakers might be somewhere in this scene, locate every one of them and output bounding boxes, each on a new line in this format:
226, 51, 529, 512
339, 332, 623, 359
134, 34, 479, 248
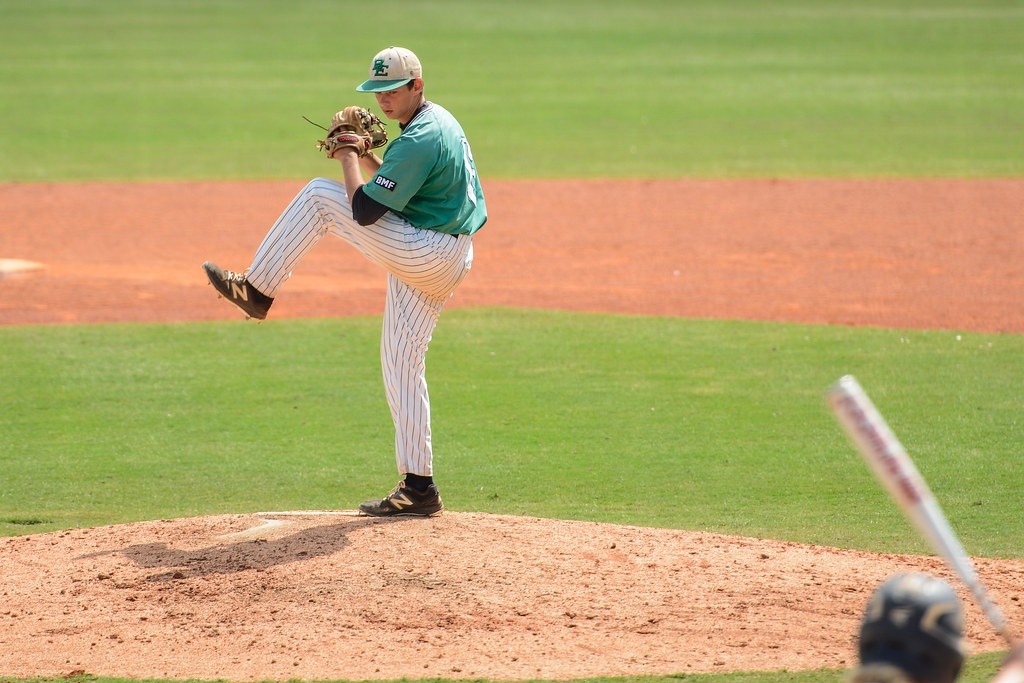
202, 261, 274, 320
358, 479, 444, 517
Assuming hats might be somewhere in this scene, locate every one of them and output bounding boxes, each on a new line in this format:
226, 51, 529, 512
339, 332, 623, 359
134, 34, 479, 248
355, 46, 423, 92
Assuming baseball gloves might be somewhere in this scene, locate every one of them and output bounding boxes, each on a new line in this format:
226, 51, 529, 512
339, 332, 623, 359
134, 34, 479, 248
302, 105, 389, 161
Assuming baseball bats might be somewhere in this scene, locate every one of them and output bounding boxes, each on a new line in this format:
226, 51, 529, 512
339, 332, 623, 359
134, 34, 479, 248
823, 371, 1020, 657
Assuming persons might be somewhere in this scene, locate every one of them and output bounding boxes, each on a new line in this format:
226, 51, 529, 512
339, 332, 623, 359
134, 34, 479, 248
203, 45, 487, 517
843, 572, 1024, 683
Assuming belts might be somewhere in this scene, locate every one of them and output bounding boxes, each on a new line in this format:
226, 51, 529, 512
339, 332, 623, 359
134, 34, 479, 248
450, 233, 459, 238
